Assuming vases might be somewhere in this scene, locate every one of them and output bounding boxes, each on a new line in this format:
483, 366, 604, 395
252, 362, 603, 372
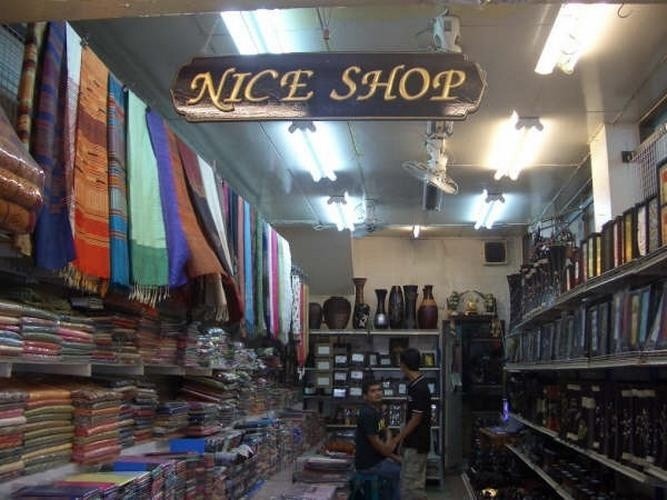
308, 278, 438, 329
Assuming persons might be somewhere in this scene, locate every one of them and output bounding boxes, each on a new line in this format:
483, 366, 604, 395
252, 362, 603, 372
354, 380, 402, 500
386, 347, 431, 500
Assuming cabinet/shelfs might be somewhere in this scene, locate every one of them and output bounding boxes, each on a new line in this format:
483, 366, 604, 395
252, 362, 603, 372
502, 245, 667, 500
305, 331, 444, 488
